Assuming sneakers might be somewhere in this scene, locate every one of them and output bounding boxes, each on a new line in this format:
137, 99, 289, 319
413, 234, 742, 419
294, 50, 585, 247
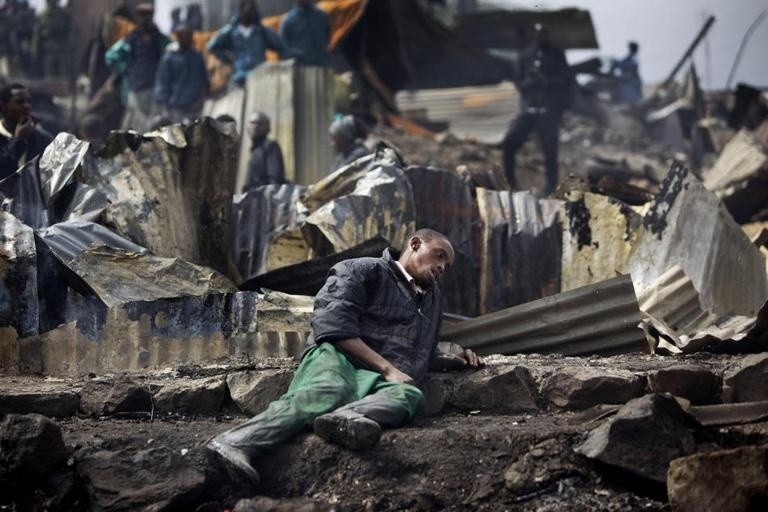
297, 413, 385, 449
198, 435, 265, 490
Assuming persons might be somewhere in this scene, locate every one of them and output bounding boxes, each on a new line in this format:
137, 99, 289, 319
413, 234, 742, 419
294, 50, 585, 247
206, 228, 485, 490
609, 43, 641, 103
0, 83, 53, 179
327, 113, 371, 168
495, 21, 580, 198
242, 112, 286, 192
104, 0, 330, 138
